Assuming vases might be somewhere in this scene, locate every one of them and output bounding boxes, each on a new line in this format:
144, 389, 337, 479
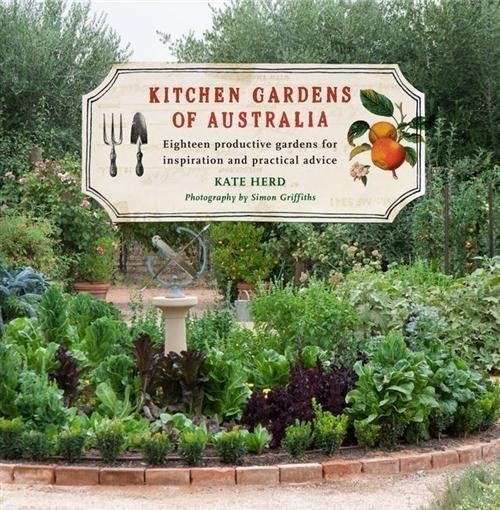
71, 284, 108, 299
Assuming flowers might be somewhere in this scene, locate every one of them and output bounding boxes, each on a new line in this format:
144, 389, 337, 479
77, 236, 119, 284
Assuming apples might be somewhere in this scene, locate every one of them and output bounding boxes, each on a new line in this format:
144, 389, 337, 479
369, 122, 398, 144
372, 138, 405, 169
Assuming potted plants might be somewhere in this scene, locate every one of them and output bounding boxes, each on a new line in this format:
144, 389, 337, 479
232, 241, 275, 298
